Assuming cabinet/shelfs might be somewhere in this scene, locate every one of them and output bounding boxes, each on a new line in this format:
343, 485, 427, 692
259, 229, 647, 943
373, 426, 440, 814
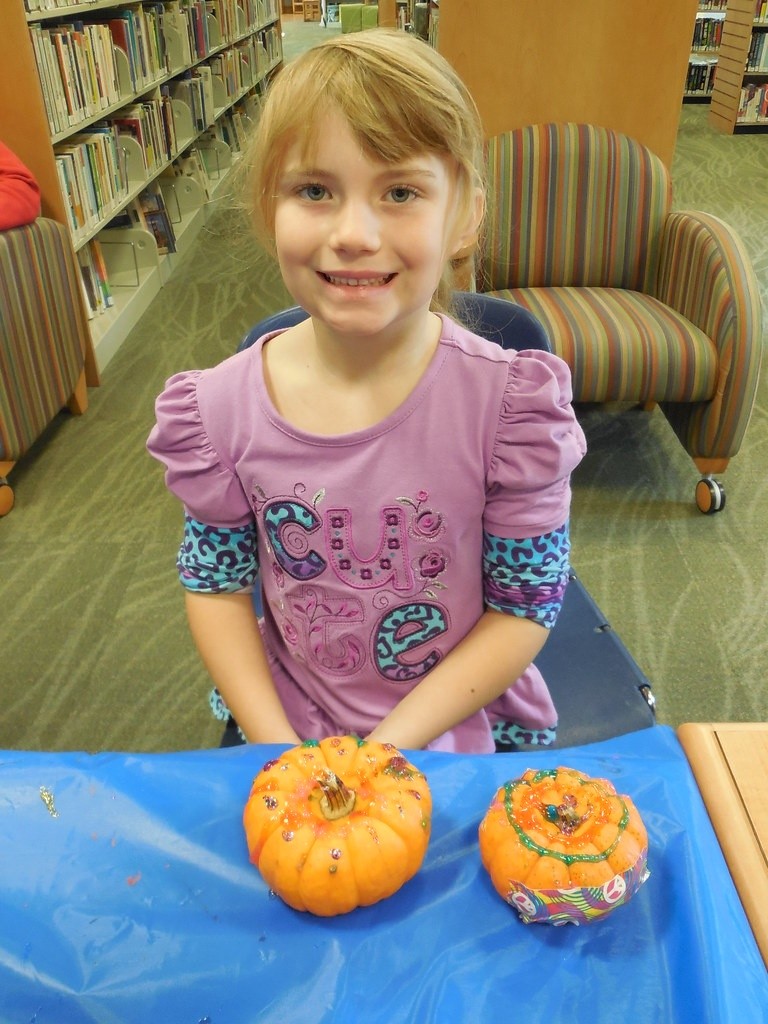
375, 0, 767, 164
1, 0, 288, 387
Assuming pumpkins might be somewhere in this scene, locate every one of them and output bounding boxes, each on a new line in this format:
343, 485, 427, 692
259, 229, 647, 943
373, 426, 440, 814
244, 737, 646, 923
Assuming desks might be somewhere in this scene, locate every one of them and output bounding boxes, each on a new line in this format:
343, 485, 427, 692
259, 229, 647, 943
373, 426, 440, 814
0, 721, 767, 1024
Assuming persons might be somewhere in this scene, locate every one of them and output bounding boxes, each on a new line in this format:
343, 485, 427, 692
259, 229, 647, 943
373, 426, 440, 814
146, 26, 588, 767
0, 140, 42, 233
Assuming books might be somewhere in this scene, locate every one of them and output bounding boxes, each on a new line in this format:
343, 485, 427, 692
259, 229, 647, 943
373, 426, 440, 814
682, 0, 768, 125
26, 0, 282, 326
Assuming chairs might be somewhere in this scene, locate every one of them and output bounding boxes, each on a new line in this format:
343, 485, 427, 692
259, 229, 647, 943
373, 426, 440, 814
452, 118, 764, 516
1, 215, 93, 521
216, 281, 660, 752
